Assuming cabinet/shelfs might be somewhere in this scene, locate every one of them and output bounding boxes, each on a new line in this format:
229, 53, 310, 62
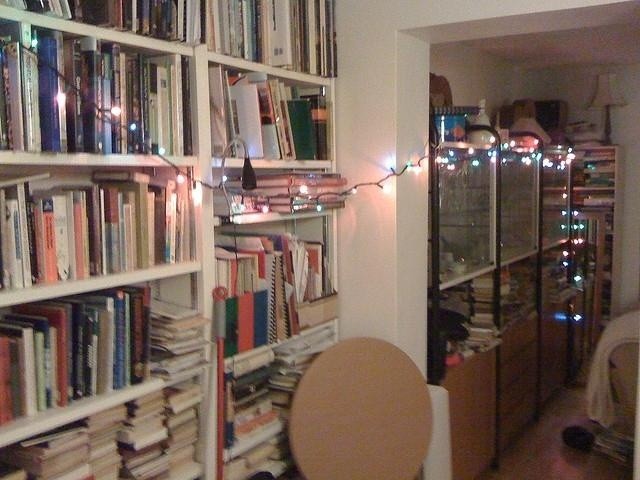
0, 7, 208, 480
205, 43, 341, 479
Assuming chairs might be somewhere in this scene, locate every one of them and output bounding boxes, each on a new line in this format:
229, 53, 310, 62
286, 332, 436, 480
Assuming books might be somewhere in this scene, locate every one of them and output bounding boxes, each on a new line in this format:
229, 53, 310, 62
210, 66, 332, 161
2, 383, 205, 478
0, 169, 192, 291
427, 144, 615, 382
2, 21, 194, 155
2, 284, 210, 425
591, 422, 634, 469
212, 174, 351, 480
2, 0, 338, 79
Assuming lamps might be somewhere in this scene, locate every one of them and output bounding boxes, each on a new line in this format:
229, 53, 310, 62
587, 72, 627, 143
221, 138, 256, 221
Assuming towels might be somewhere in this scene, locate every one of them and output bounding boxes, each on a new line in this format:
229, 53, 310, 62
581, 309, 638, 429
